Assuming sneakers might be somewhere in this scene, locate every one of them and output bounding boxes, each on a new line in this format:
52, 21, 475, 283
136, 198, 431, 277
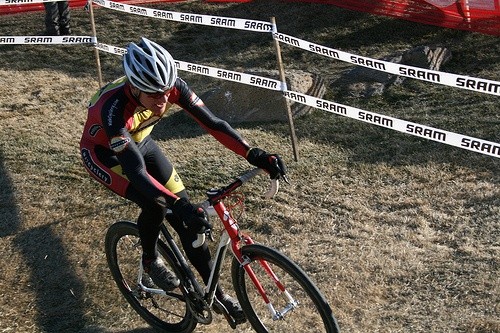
141, 256, 180, 290
213, 294, 247, 319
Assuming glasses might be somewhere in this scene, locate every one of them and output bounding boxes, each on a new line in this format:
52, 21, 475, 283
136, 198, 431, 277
142, 86, 175, 99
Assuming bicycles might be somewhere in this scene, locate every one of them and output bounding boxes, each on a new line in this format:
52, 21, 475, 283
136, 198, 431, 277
103, 155, 339, 333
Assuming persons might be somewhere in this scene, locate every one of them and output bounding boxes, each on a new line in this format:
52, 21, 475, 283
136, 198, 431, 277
79, 36, 286, 323
43, 0, 71, 36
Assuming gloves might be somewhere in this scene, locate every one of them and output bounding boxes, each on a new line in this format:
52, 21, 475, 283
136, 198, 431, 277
172, 197, 213, 235
247, 146, 288, 180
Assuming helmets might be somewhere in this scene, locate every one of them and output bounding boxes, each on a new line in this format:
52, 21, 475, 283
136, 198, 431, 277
121, 36, 177, 95
